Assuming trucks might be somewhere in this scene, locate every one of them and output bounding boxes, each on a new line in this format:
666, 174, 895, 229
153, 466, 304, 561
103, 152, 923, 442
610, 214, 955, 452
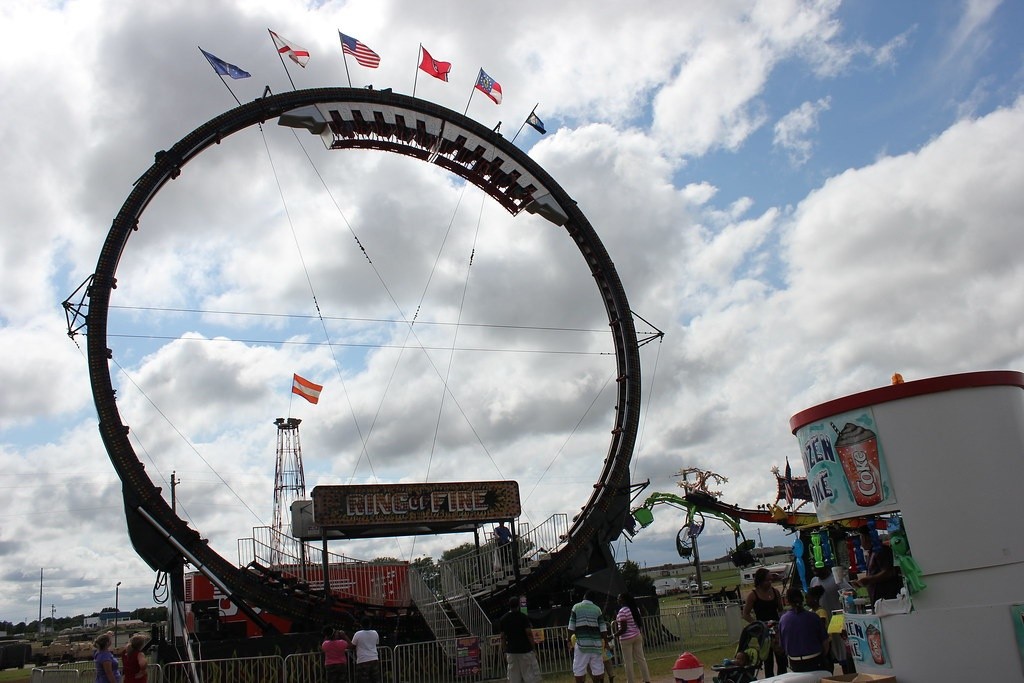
653, 578, 689, 597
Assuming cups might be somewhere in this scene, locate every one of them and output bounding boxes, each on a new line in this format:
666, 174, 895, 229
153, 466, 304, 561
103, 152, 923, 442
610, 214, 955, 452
867, 634, 884, 664
832, 567, 842, 584
857, 574, 866, 581
671, 651, 704, 683
834, 435, 884, 507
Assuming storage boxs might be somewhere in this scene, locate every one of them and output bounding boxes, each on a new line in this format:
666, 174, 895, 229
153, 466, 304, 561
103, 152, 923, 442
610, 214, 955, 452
820, 672, 897, 683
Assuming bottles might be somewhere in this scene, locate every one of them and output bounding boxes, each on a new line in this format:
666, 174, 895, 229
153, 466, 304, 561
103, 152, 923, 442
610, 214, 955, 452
854, 599, 866, 614
724, 656, 729, 668
846, 593, 855, 613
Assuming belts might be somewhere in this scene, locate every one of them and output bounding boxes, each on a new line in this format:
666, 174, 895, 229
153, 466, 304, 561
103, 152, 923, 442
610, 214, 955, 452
788, 652, 822, 661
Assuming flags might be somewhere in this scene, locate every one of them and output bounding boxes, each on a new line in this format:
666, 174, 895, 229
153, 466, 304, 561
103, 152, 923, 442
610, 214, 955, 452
418, 46, 451, 82
268, 29, 310, 68
475, 67, 503, 104
339, 31, 381, 69
526, 112, 547, 135
291, 373, 323, 404
199, 48, 252, 79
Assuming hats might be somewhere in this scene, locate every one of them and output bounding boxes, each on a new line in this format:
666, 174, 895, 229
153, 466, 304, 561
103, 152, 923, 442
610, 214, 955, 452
858, 524, 876, 535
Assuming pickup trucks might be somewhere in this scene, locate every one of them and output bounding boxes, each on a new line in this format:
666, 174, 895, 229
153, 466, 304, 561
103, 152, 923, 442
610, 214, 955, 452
685, 583, 699, 594
702, 581, 713, 590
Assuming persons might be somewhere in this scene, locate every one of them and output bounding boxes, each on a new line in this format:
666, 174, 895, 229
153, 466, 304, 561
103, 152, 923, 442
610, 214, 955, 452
713, 524, 893, 683
567, 589, 653, 683
93, 631, 150, 683
497, 596, 543, 683
321, 625, 354, 683
348, 619, 380, 683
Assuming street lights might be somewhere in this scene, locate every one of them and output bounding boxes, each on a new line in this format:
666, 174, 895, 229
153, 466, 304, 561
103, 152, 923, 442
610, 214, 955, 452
114, 582, 121, 647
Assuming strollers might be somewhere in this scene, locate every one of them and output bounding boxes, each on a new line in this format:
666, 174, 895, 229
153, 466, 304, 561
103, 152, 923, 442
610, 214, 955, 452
710, 621, 771, 683
